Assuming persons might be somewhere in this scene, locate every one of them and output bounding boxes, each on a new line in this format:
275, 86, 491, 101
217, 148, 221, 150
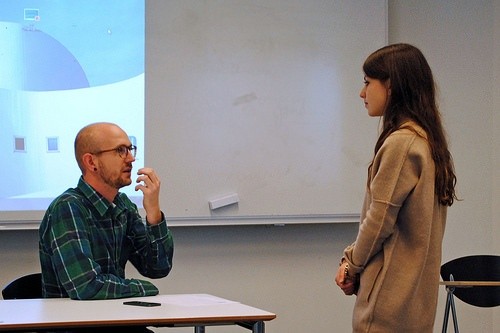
334, 41, 465, 333
38, 121, 175, 332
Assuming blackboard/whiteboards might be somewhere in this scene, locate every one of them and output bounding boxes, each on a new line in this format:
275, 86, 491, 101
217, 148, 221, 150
0, 0, 392, 230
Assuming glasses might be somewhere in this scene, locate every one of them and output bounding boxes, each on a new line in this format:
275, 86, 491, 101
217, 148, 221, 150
90, 144, 136, 161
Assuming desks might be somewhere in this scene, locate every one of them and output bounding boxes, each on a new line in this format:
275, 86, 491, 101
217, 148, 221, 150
0, 294, 277, 333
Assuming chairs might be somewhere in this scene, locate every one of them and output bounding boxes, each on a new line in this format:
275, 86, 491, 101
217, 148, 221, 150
437, 255, 500, 333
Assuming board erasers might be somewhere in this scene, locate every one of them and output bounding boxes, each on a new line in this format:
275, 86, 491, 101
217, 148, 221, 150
209, 191, 239, 210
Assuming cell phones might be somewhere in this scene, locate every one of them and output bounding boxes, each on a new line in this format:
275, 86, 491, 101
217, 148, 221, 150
124, 300, 160, 308
